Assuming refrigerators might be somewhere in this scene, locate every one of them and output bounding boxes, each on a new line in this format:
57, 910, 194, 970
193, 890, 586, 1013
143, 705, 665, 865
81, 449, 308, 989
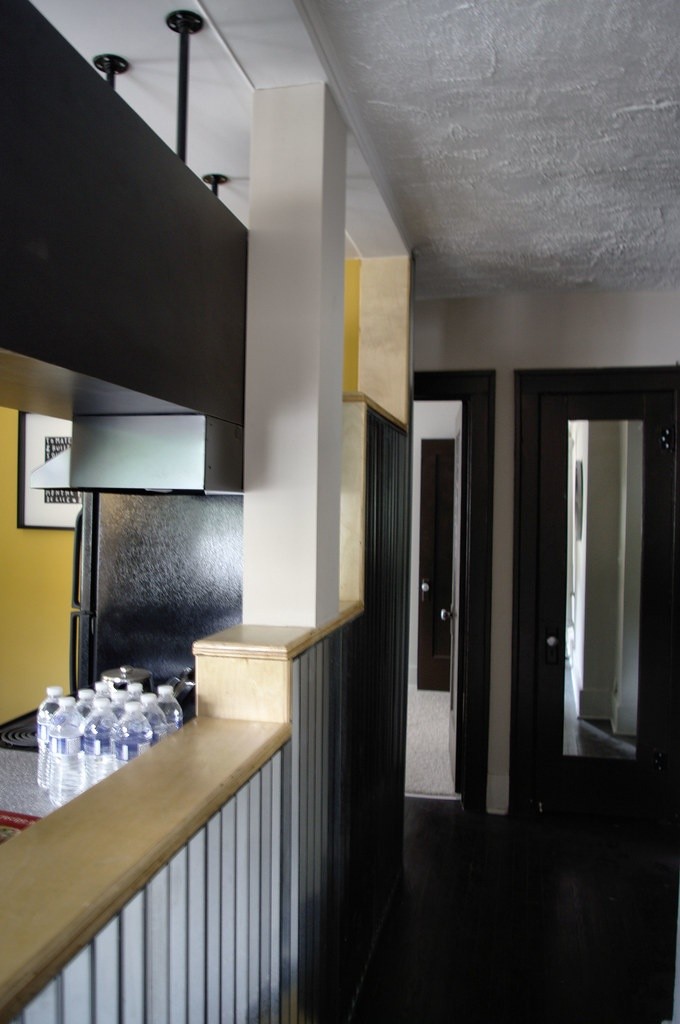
71, 489, 242, 726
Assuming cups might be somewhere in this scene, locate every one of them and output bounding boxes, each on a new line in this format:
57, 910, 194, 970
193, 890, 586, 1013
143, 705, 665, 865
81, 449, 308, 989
100, 665, 154, 694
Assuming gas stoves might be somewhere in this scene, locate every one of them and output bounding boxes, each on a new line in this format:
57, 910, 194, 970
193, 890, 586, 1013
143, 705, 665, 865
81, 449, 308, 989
0, 697, 58, 820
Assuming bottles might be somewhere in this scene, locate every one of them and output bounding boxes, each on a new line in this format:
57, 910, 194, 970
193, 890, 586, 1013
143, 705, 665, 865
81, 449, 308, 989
35, 681, 183, 806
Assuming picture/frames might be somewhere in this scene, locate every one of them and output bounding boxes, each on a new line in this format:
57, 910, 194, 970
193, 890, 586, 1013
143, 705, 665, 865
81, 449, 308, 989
16, 410, 81, 530
